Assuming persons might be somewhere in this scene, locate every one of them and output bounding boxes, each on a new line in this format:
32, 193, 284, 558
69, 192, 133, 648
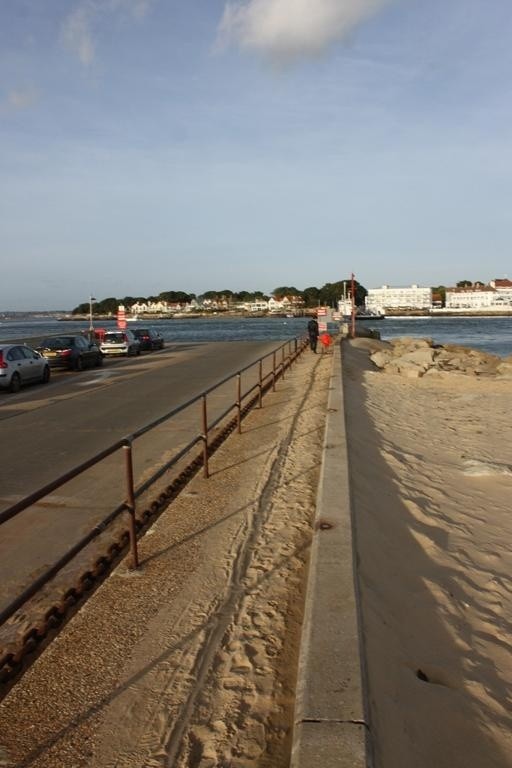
308, 315, 319, 354
318, 330, 333, 353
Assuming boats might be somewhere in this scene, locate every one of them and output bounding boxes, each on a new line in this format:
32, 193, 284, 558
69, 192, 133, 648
348, 310, 386, 320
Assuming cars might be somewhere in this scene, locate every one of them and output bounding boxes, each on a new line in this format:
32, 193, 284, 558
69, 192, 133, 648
97, 328, 142, 358
128, 326, 165, 353
34, 333, 106, 373
0, 343, 51, 393
